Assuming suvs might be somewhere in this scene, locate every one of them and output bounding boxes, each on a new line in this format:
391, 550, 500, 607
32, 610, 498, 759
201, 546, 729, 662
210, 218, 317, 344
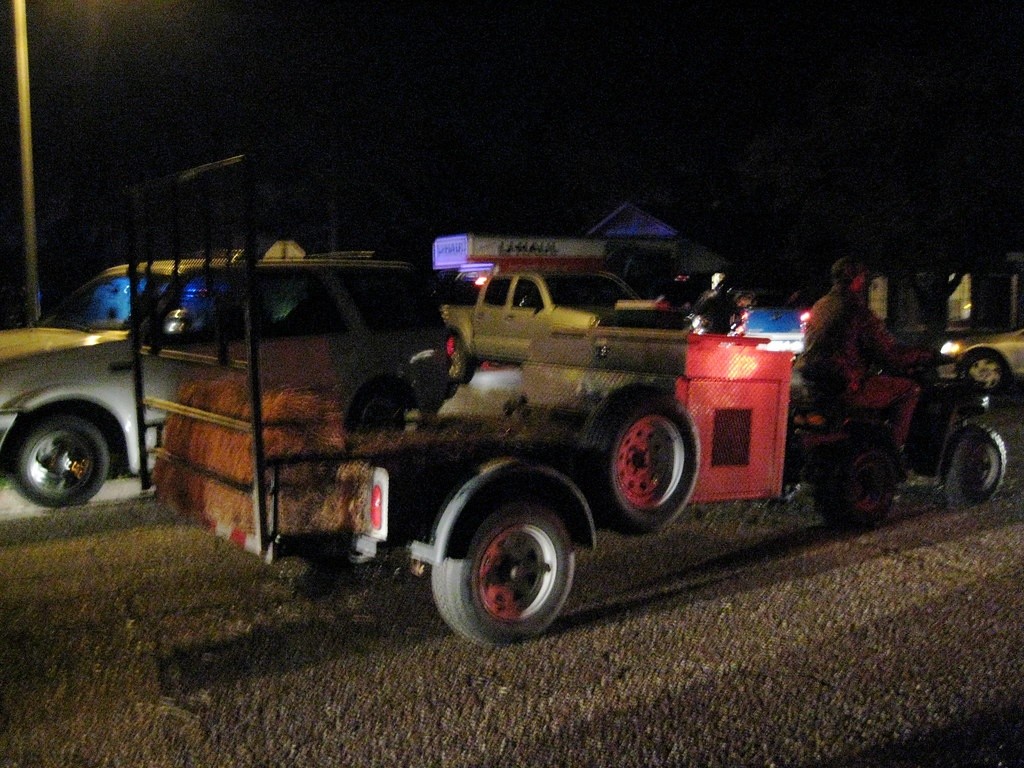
1, 253, 460, 510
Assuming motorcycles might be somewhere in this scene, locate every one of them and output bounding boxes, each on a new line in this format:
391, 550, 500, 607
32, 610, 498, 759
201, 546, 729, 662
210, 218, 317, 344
793, 354, 1007, 535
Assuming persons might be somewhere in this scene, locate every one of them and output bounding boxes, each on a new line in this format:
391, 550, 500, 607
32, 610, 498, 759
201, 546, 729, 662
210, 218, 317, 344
693, 273, 745, 333
799, 253, 933, 483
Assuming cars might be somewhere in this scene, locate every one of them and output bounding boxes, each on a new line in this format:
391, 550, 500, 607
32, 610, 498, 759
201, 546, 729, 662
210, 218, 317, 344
938, 321, 1024, 394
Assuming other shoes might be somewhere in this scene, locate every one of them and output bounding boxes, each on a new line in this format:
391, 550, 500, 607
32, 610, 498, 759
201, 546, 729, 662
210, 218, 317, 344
884, 436, 914, 470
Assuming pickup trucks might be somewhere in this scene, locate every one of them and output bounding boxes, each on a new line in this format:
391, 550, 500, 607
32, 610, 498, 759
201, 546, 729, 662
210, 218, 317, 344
442, 267, 673, 388
724, 304, 810, 359
